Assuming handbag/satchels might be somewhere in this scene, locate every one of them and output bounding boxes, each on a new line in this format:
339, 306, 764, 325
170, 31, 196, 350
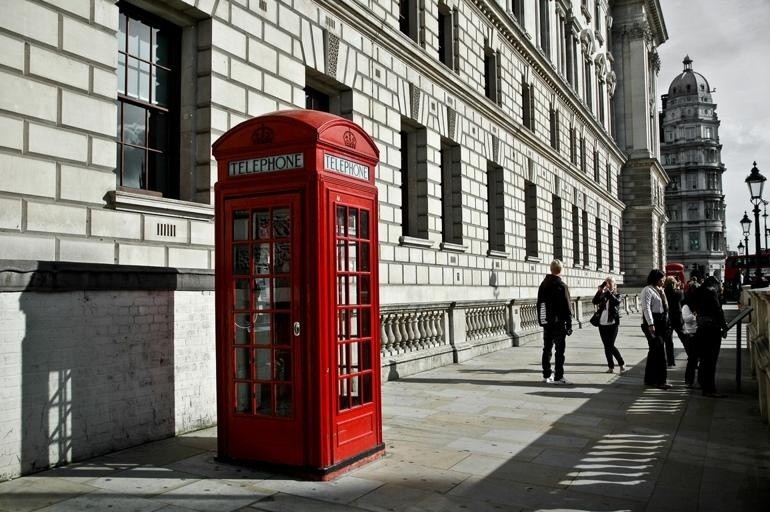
590, 309, 601, 327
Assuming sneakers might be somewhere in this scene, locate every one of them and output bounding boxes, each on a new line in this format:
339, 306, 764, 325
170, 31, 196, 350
685, 384, 700, 388
620, 362, 626, 374
553, 377, 575, 385
607, 367, 614, 373
544, 375, 554, 383
702, 390, 721, 398
644, 380, 673, 387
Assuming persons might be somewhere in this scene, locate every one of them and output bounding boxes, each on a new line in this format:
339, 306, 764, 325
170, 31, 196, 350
664, 275, 700, 369
590, 276, 627, 374
679, 284, 703, 387
537, 259, 574, 385
675, 275, 704, 290
697, 276, 729, 399
640, 268, 673, 388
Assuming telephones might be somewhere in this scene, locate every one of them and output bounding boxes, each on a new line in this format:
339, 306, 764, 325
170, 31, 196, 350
238, 245, 272, 304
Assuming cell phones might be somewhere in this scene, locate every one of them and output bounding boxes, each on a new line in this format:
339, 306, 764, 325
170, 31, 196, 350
601, 281, 607, 289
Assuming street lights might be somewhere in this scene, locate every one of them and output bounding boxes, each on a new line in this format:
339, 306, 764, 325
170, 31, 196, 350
737, 160, 767, 288
